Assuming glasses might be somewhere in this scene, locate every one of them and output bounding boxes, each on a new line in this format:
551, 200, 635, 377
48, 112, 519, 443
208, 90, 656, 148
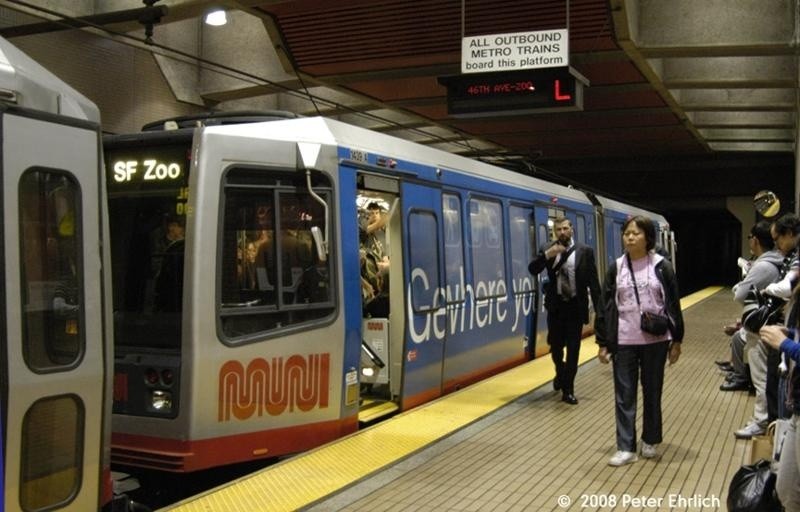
771, 232, 781, 245
747, 234, 757, 240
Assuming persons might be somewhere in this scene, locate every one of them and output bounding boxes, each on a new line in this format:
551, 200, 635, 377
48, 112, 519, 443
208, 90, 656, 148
528, 216, 601, 405
594, 215, 684, 466
237, 202, 389, 320
714, 214, 800, 512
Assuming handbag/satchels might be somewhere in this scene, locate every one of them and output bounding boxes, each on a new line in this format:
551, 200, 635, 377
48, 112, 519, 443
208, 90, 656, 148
740, 284, 782, 335
541, 242, 575, 296
637, 309, 669, 337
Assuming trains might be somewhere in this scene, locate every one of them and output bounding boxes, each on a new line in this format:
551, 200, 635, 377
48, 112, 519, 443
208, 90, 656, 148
0, 34, 679, 511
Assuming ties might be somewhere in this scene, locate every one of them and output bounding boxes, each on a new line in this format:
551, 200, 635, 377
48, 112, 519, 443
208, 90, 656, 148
558, 242, 573, 303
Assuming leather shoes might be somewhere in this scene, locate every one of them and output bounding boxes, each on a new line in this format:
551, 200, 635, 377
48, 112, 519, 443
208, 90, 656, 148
553, 365, 564, 391
562, 392, 580, 404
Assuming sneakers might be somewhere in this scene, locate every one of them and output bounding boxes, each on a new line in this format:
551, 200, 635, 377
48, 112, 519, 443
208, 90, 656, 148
714, 355, 752, 393
733, 415, 766, 440
641, 436, 658, 459
608, 447, 639, 466
